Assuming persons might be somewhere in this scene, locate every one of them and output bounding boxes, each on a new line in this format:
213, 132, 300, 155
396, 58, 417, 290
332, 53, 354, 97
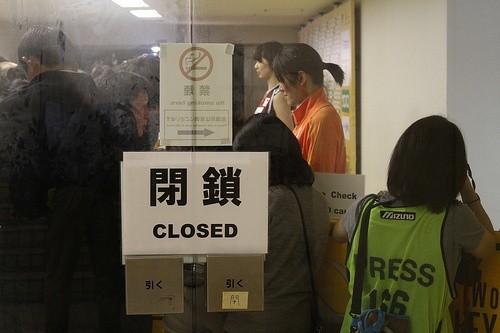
232, 112, 331, 333
254, 41, 295, 132
272, 43, 347, 175
1, 55, 30, 98
92, 54, 167, 152
333, 115, 496, 333
0, 26, 125, 333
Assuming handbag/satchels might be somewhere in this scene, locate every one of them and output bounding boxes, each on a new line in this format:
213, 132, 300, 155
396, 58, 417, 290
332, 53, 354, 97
350, 309, 409, 333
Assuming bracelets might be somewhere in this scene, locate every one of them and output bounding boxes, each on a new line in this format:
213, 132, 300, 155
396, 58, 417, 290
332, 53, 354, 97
467, 198, 481, 203
